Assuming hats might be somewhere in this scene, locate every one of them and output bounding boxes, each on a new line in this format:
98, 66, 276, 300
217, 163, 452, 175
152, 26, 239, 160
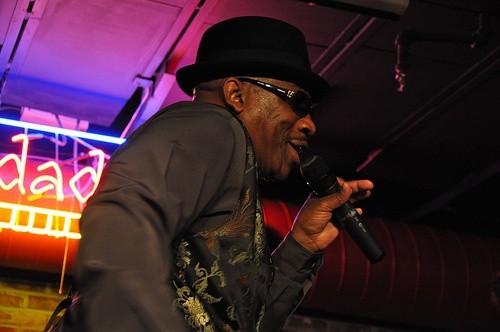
175, 14, 331, 104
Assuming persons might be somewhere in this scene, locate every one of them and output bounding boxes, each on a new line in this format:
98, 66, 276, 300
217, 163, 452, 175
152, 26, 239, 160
37, 19, 379, 332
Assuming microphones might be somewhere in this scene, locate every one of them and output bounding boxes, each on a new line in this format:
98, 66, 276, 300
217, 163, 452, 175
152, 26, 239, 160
300, 153, 385, 264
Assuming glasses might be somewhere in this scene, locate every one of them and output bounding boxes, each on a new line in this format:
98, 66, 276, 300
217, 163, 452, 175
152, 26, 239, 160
236, 77, 313, 118
